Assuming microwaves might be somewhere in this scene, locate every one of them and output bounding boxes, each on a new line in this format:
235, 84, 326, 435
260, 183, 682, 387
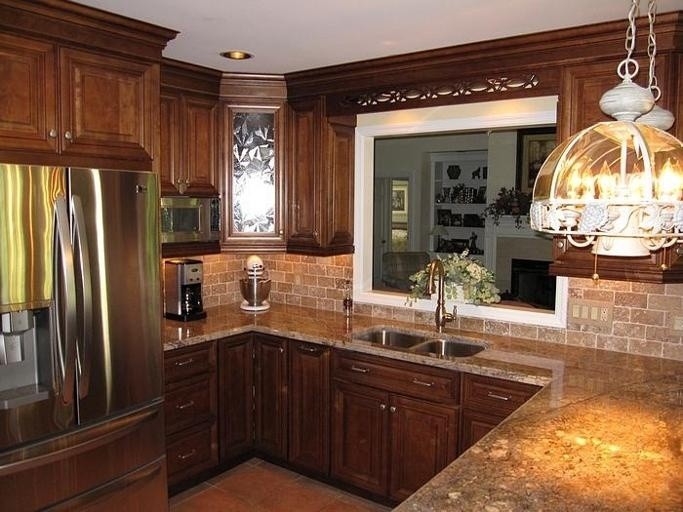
160, 196, 220, 244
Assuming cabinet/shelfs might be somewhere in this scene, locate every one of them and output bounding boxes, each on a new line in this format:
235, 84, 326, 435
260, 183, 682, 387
159, 84, 219, 196
250, 330, 328, 480
431, 157, 487, 258
222, 101, 286, 251
1, 4, 159, 160
460, 372, 541, 451
330, 351, 460, 508
164, 332, 250, 487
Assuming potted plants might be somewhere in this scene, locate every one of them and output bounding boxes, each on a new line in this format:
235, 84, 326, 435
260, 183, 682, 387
401, 249, 500, 305
483, 187, 529, 229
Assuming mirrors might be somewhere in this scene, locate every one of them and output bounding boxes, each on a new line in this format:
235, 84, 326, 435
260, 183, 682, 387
351, 96, 558, 331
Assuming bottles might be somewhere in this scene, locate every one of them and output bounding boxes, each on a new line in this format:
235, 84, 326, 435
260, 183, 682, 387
342, 280, 352, 317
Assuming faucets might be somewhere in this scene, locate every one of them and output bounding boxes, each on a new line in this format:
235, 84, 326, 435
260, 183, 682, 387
428, 259, 445, 328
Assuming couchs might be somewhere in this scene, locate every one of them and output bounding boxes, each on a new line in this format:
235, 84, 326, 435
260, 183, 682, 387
381, 250, 429, 291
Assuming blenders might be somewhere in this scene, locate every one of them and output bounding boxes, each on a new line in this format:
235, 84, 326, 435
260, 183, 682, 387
237, 255, 271, 312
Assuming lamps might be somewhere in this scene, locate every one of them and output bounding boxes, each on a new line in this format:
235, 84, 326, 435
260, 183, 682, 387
530, 1, 683, 259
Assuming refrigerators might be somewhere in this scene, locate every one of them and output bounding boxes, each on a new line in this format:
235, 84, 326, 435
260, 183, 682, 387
0, 159, 166, 512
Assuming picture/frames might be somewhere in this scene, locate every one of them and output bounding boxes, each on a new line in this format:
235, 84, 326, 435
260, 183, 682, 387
515, 129, 557, 196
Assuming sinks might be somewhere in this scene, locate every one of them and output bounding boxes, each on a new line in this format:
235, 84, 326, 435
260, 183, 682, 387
412, 340, 487, 358
358, 329, 428, 349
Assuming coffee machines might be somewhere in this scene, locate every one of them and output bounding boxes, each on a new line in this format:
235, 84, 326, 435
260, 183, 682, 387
164, 259, 208, 323
